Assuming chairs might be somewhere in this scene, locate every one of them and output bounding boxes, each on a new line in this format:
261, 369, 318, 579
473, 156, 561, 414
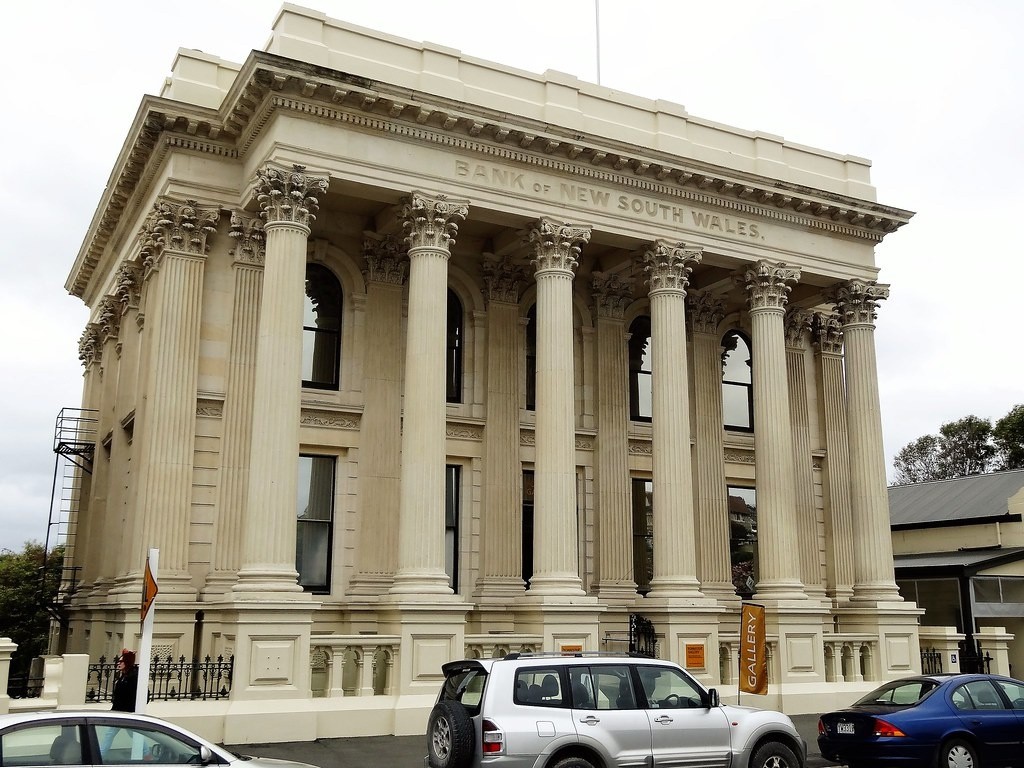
975, 687, 999, 709
518, 675, 595, 707
50, 736, 81, 763
615, 677, 634, 708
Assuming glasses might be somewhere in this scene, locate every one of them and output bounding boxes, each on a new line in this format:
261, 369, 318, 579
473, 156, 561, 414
118, 660, 124, 663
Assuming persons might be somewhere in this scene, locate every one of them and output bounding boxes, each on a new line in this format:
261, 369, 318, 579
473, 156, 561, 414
99, 652, 150, 761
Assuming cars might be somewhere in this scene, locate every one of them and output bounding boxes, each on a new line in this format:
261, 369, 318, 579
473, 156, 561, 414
0, 713, 319, 768
818, 672, 1024, 768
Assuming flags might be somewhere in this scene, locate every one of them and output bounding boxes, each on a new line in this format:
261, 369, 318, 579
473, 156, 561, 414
740, 604, 768, 695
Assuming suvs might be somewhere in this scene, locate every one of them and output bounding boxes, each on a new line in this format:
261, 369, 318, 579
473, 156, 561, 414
423, 650, 807, 768
